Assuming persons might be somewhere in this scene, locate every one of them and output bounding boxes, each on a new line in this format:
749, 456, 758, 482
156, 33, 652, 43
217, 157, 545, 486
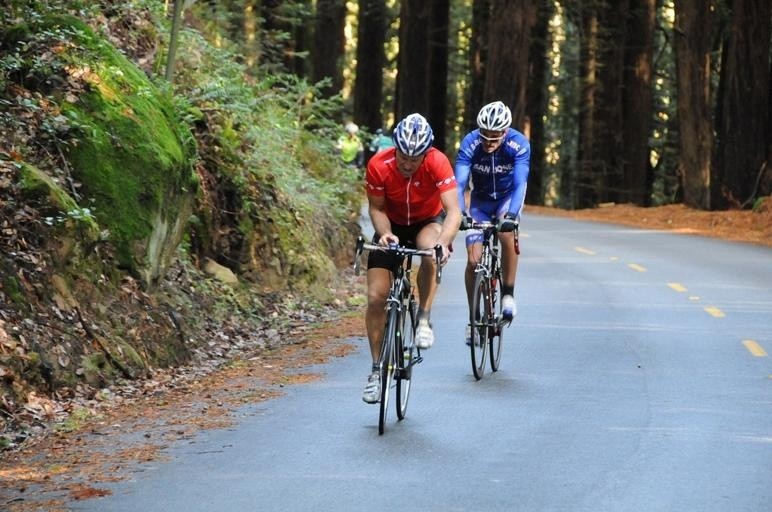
359, 111, 460, 404
331, 123, 389, 169
455, 100, 533, 345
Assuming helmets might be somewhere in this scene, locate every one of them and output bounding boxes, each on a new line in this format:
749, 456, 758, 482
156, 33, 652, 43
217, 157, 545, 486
476, 100, 513, 132
393, 113, 435, 159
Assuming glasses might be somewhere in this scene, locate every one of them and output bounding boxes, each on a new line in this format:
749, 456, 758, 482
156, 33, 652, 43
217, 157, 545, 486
498, 213, 517, 232
477, 132, 506, 143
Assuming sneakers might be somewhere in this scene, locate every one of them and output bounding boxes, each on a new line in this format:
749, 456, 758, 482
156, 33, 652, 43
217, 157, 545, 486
362, 373, 381, 404
465, 326, 479, 346
414, 318, 434, 349
501, 294, 517, 320
